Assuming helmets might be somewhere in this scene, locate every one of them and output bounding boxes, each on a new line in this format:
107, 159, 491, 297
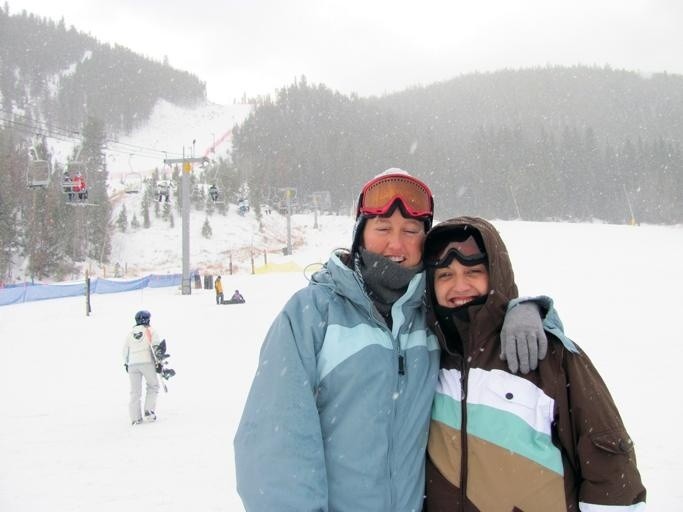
135, 311, 150, 325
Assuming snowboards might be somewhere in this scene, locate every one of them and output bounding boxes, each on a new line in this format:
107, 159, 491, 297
222, 300, 244, 305
144, 329, 175, 392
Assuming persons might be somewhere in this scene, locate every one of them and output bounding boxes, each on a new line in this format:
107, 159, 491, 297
214, 275, 225, 304
159, 191, 169, 202
422, 216, 648, 512
210, 184, 218, 201
233, 166, 580, 512
73, 171, 88, 203
62, 171, 75, 202
232, 290, 243, 304
123, 309, 165, 426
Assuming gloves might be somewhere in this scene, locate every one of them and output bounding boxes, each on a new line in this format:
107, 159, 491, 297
498, 303, 548, 377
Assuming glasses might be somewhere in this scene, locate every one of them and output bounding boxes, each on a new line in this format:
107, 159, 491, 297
355, 174, 435, 234
423, 230, 488, 268
